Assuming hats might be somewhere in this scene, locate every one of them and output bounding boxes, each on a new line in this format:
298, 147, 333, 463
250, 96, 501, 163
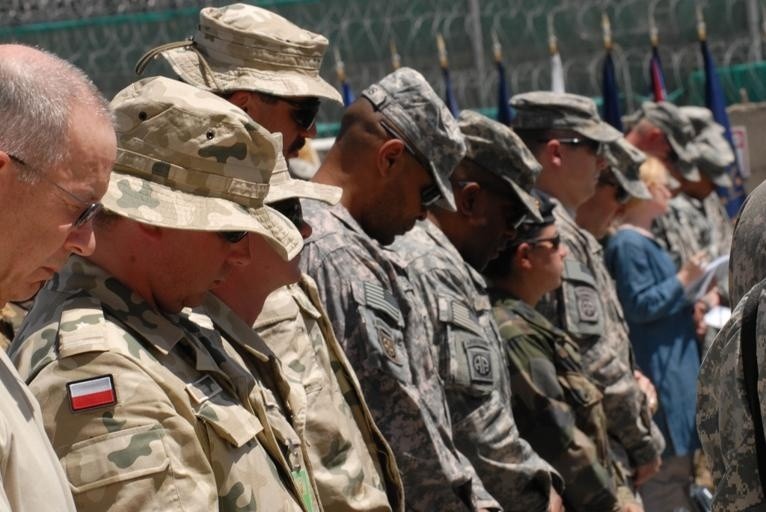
639, 101, 737, 192
524, 189, 556, 225
602, 139, 648, 198
509, 88, 623, 144
93, 74, 306, 262
157, 1, 346, 105
261, 131, 342, 202
362, 66, 463, 216
455, 104, 547, 225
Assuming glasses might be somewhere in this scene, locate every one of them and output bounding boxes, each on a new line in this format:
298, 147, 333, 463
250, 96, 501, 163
289, 98, 325, 128
558, 136, 603, 157
5, 150, 103, 228
220, 231, 248, 243
494, 179, 526, 232
378, 117, 442, 207
533, 230, 563, 247
603, 175, 630, 203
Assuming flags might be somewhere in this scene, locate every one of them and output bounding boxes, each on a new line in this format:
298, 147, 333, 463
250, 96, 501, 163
598, 47, 626, 136
642, 45, 670, 106
434, 64, 461, 120
491, 64, 515, 127
698, 40, 746, 221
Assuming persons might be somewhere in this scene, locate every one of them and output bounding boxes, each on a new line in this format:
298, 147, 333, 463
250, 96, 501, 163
148, 0, 405, 512
482, 87, 765, 512
1, 39, 123, 511
295, 66, 503, 512
206, 128, 344, 511
382, 108, 570, 510
0, 73, 325, 510
